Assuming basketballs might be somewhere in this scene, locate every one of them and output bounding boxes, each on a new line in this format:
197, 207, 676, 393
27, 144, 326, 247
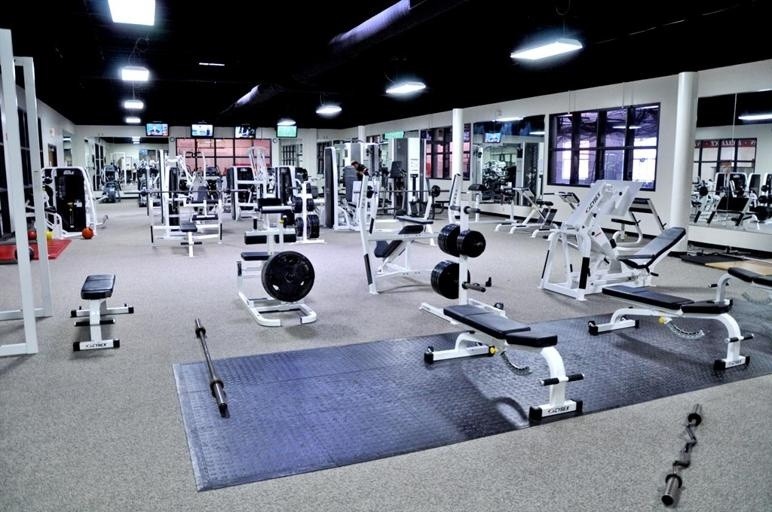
82, 228, 93, 239
28, 230, 36, 240
47, 231, 53, 239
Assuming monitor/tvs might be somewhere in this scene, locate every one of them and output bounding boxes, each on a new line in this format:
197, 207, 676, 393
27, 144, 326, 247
145, 122, 169, 136
276, 125, 298, 138
191, 124, 214, 139
484, 133, 501, 143
234, 126, 256, 138
382, 131, 405, 140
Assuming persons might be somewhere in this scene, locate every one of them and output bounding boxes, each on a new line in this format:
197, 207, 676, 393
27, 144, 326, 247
351, 160, 369, 181
507, 161, 516, 187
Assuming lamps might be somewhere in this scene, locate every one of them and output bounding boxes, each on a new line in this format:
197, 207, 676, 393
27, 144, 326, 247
315, 104, 341, 117
385, 81, 426, 96
509, 37, 583, 61
118, 65, 151, 126
278, 118, 296, 127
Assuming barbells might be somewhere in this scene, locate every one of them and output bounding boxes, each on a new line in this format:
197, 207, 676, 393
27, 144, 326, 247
123, 187, 246, 197
354, 186, 450, 198
195, 318, 228, 411
694, 186, 749, 196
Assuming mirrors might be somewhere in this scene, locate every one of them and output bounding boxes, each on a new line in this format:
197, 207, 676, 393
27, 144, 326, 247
689, 85, 772, 236
